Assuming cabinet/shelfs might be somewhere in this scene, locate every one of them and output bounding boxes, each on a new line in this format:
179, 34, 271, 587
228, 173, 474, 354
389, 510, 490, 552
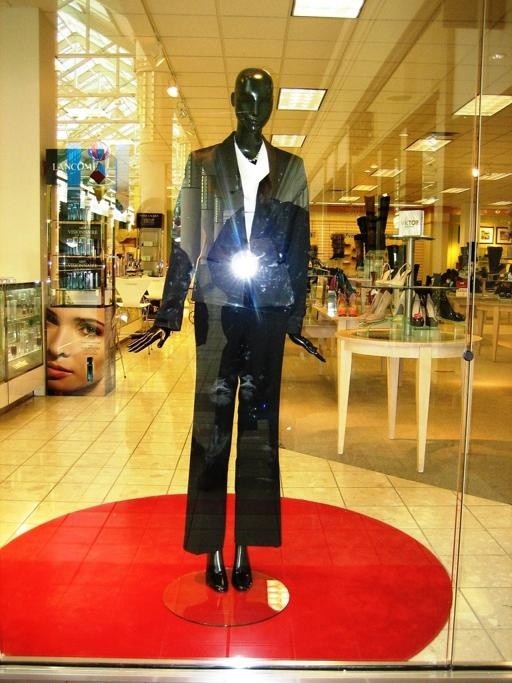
121, 209, 167, 276
0, 146, 121, 414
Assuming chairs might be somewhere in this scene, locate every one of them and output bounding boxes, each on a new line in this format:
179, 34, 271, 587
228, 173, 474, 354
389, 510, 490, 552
113, 280, 153, 355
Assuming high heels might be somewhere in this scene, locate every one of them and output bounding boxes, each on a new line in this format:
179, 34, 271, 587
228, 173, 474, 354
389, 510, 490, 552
375, 263, 394, 286
359, 289, 465, 327
387, 263, 412, 286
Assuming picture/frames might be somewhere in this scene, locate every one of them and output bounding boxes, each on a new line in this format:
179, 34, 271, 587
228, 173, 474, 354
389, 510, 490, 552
478, 224, 512, 246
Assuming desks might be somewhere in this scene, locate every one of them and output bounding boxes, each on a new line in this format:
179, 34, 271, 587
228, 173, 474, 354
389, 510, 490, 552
474, 298, 512, 361
331, 329, 485, 473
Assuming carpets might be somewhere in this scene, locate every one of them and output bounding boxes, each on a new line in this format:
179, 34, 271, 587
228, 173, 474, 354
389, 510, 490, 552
1, 493, 452, 666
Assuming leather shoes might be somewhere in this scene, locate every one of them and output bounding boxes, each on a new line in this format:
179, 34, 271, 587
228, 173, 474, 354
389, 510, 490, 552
349, 293, 359, 317
337, 294, 346, 316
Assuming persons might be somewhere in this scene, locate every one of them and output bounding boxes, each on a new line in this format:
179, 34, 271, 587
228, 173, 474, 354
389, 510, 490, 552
124, 65, 329, 597
45, 306, 107, 396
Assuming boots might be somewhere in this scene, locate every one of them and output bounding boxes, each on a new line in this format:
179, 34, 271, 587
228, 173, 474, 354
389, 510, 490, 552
330, 234, 344, 260
366, 196, 376, 258
357, 216, 366, 235
354, 235, 364, 270
376, 195, 390, 258
